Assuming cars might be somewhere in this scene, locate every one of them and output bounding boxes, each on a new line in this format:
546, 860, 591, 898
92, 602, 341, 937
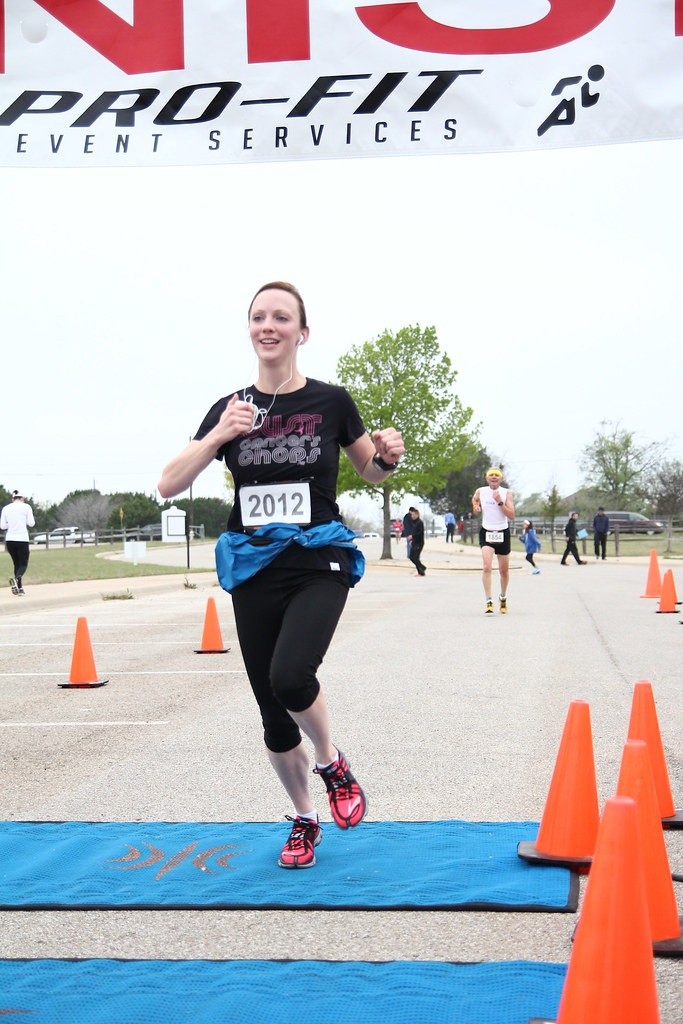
363, 534, 379, 537
34, 527, 95, 545
605, 512, 665, 535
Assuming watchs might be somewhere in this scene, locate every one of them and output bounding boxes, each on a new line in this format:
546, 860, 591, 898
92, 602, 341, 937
373, 452, 398, 472
498, 502, 503, 506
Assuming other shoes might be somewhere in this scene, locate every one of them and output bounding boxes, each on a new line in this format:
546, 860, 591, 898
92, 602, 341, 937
414, 572, 424, 576
578, 561, 587, 565
532, 569, 539, 574
561, 562, 567, 566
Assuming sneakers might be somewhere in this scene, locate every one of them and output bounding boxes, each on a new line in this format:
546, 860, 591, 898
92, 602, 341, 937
500, 594, 507, 615
8, 577, 19, 595
310, 744, 366, 830
19, 589, 25, 596
277, 815, 321, 870
485, 600, 493, 614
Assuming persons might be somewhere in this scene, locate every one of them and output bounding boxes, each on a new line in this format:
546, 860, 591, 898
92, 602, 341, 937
159, 281, 406, 866
0, 489, 35, 595
404, 507, 415, 558
407, 510, 426, 576
561, 512, 587, 565
472, 467, 515, 614
445, 510, 455, 543
518, 520, 541, 574
593, 507, 608, 560
393, 519, 402, 543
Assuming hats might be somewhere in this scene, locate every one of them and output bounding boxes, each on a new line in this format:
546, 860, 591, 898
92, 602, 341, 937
12, 490, 24, 497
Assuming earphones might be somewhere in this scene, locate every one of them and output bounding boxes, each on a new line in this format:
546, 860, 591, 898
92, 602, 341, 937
298, 334, 304, 345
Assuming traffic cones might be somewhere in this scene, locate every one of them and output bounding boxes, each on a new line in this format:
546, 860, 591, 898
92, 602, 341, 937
657, 568, 683, 604
639, 549, 663, 599
614, 741, 683, 925
627, 681, 683, 831
57, 617, 108, 688
570, 740, 683, 959
526, 796, 661, 1024
516, 699, 598, 867
193, 597, 231, 653
655, 572, 680, 613
